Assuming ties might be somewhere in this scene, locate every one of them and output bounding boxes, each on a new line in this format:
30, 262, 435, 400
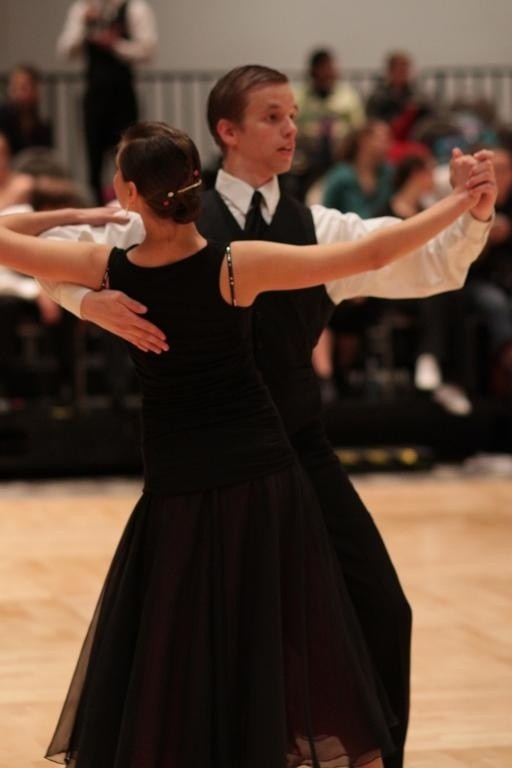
244, 190, 270, 241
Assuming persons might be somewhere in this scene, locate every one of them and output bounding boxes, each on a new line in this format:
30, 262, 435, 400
294, 49, 512, 460
2, 0, 160, 472
32, 62, 501, 768
0, 120, 484, 768
